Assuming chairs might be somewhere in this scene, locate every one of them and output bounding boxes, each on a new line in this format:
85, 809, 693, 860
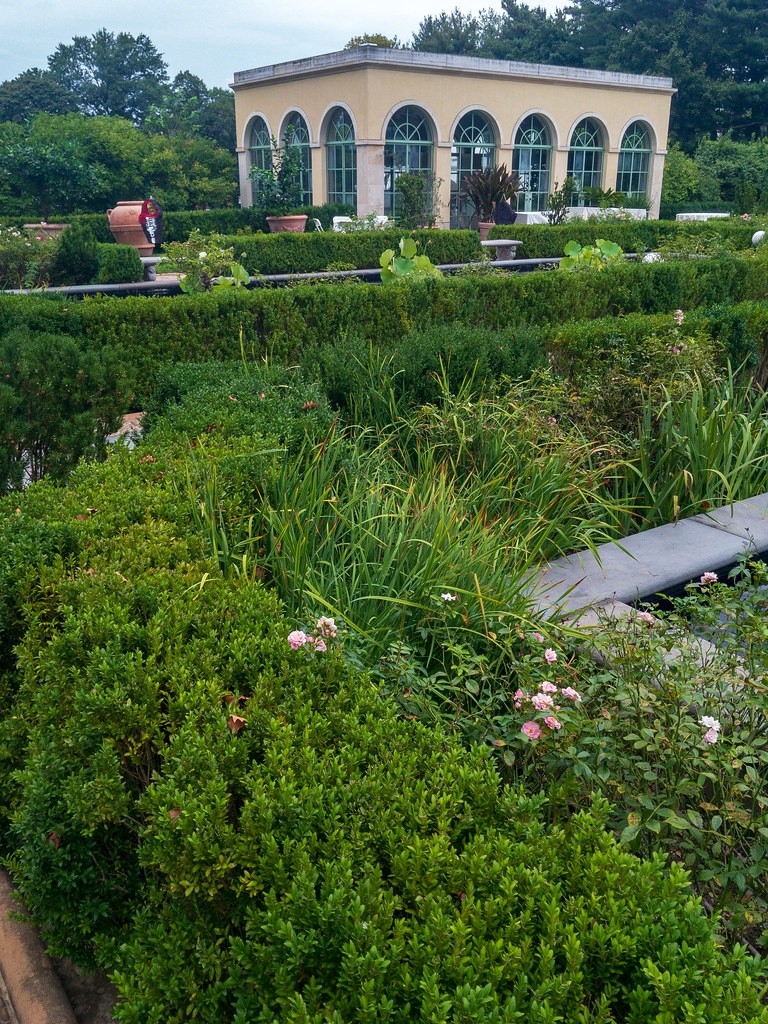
313, 218, 324, 233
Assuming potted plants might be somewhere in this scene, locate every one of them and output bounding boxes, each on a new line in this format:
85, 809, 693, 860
455, 162, 527, 241
246, 123, 308, 234
